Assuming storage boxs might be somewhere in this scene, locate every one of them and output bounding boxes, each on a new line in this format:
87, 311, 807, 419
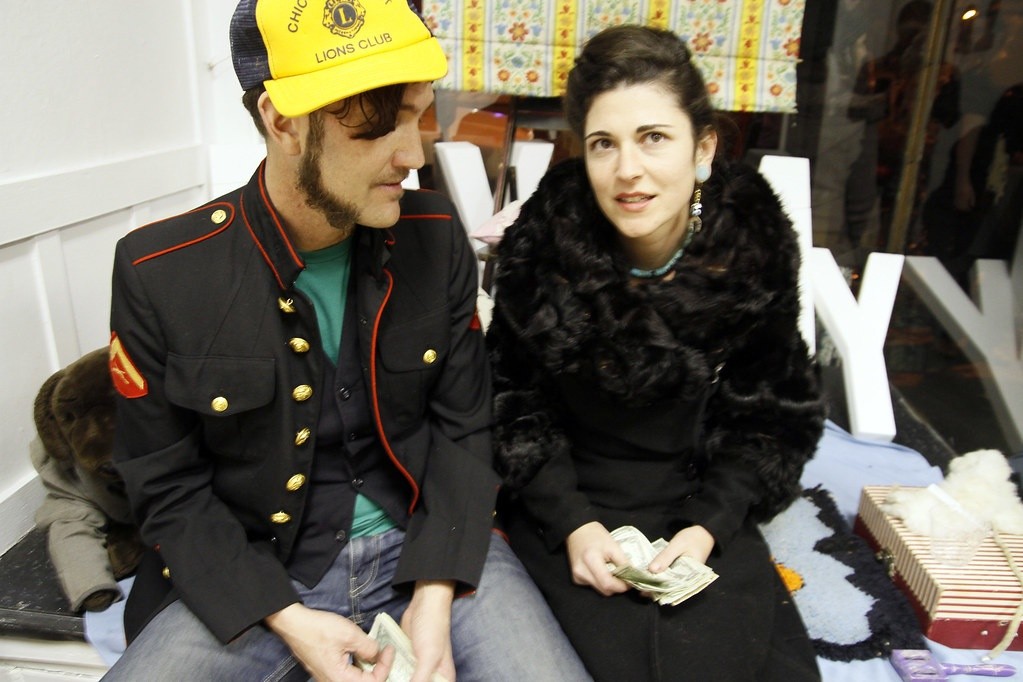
853, 485, 1023, 651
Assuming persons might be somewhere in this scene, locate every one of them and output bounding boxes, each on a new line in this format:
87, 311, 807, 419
95, 0, 499, 682
484, 25, 826, 682
841, 1, 962, 270
969, 83, 1023, 262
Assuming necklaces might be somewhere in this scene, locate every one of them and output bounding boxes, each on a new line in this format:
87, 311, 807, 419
630, 221, 694, 277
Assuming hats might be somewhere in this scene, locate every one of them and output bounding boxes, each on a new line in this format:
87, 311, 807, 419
229, 0, 451, 118
